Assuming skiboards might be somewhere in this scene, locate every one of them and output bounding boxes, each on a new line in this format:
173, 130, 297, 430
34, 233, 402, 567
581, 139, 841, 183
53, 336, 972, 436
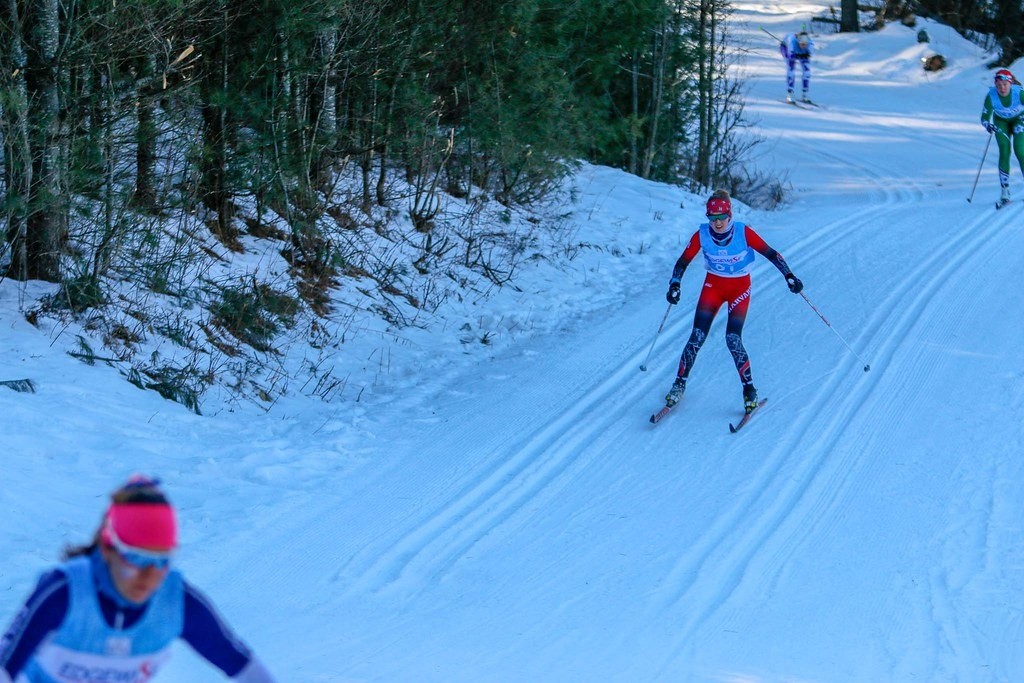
995, 198, 1024, 211
649, 396, 768, 435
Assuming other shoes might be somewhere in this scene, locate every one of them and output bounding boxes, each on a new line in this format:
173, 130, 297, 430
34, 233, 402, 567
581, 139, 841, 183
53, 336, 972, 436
800, 97, 811, 103
787, 96, 795, 104
1000, 190, 1010, 203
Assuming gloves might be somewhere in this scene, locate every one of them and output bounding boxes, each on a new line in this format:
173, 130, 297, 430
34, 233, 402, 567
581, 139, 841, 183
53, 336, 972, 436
785, 272, 803, 294
987, 123, 998, 135
666, 282, 681, 305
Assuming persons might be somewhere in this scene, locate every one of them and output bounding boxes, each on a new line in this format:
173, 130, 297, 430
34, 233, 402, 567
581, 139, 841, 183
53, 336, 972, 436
0, 473, 277, 683
663, 190, 805, 415
780, 32, 816, 105
981, 69, 1024, 205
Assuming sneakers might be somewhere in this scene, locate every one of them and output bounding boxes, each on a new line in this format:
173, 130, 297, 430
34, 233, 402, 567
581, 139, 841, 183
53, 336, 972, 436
743, 389, 757, 414
665, 379, 685, 406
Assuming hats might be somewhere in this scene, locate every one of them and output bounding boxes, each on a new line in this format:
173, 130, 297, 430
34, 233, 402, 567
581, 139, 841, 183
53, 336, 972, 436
707, 189, 732, 218
101, 474, 177, 556
996, 70, 1014, 83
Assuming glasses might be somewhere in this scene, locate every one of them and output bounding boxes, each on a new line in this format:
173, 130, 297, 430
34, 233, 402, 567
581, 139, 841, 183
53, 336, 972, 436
995, 75, 1008, 81
122, 548, 169, 570
706, 210, 729, 222
